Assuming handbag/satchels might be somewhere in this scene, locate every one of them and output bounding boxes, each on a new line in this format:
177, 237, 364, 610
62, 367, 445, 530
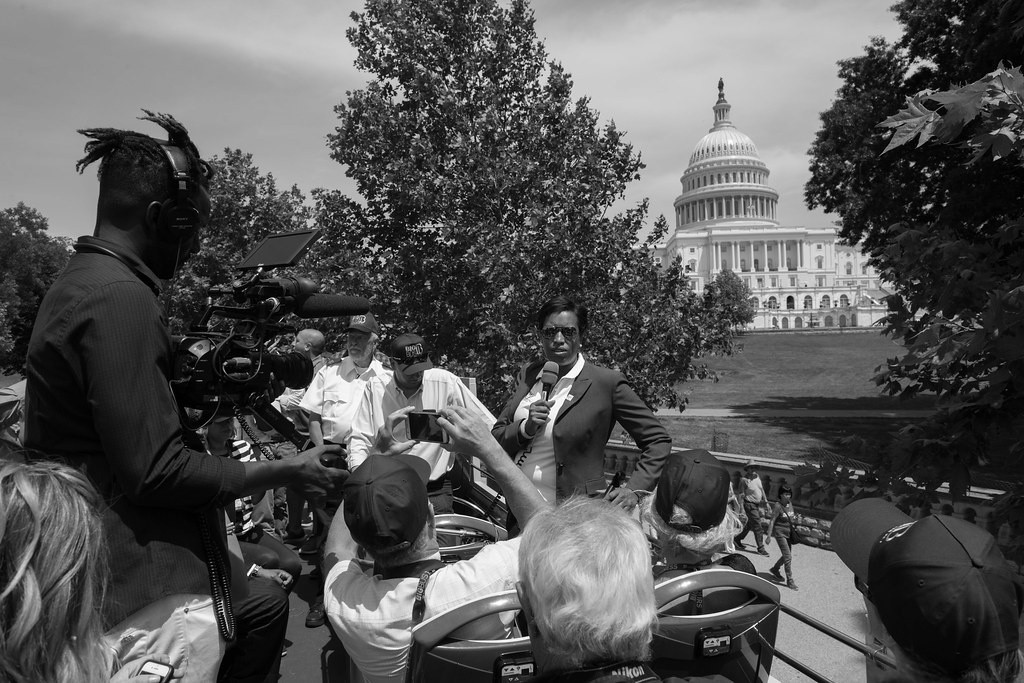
791, 525, 801, 544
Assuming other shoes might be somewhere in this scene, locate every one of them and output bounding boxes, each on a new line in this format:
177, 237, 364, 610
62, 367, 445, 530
734, 537, 746, 549
283, 525, 306, 542
299, 535, 318, 554
787, 581, 798, 590
770, 567, 785, 581
758, 549, 769, 556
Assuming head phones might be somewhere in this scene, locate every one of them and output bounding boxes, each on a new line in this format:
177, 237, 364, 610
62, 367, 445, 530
151, 137, 203, 247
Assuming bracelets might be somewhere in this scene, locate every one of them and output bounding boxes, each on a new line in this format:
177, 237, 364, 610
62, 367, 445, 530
764, 502, 769, 504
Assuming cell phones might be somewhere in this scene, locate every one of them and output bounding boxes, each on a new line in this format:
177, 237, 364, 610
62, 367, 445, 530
404, 411, 450, 444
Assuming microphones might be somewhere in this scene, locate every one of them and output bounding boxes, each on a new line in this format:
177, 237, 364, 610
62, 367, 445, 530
265, 293, 369, 319
542, 361, 560, 403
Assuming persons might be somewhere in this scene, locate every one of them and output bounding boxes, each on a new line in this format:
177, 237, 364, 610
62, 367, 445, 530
1, 314, 748, 683
765, 484, 797, 589
733, 459, 773, 557
830, 498, 1024, 683
24, 108, 353, 683
491, 297, 672, 543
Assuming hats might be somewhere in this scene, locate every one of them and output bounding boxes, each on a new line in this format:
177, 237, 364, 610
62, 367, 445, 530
341, 453, 431, 554
744, 459, 760, 471
831, 499, 1019, 677
391, 333, 433, 375
343, 311, 379, 334
656, 448, 730, 532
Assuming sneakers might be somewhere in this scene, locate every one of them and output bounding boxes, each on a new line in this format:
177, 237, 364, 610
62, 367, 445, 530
307, 601, 326, 627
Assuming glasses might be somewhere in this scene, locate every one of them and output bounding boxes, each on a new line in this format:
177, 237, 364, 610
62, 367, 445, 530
538, 327, 577, 339
393, 353, 428, 364
750, 467, 757, 469
854, 574, 876, 603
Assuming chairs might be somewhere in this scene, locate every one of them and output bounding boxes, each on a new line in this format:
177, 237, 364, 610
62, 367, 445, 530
405, 587, 538, 683
436, 514, 508, 569
646, 567, 780, 683
